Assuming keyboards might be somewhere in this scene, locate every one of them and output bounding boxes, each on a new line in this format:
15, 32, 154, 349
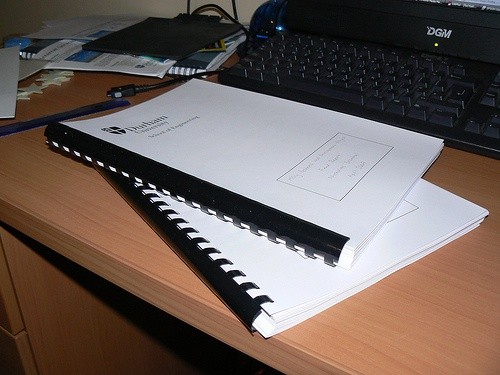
218, 29, 500, 160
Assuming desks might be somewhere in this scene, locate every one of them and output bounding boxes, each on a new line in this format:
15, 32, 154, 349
1, 32, 500, 374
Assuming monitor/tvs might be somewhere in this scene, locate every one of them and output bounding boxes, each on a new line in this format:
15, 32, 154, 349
271, 0, 500, 64
